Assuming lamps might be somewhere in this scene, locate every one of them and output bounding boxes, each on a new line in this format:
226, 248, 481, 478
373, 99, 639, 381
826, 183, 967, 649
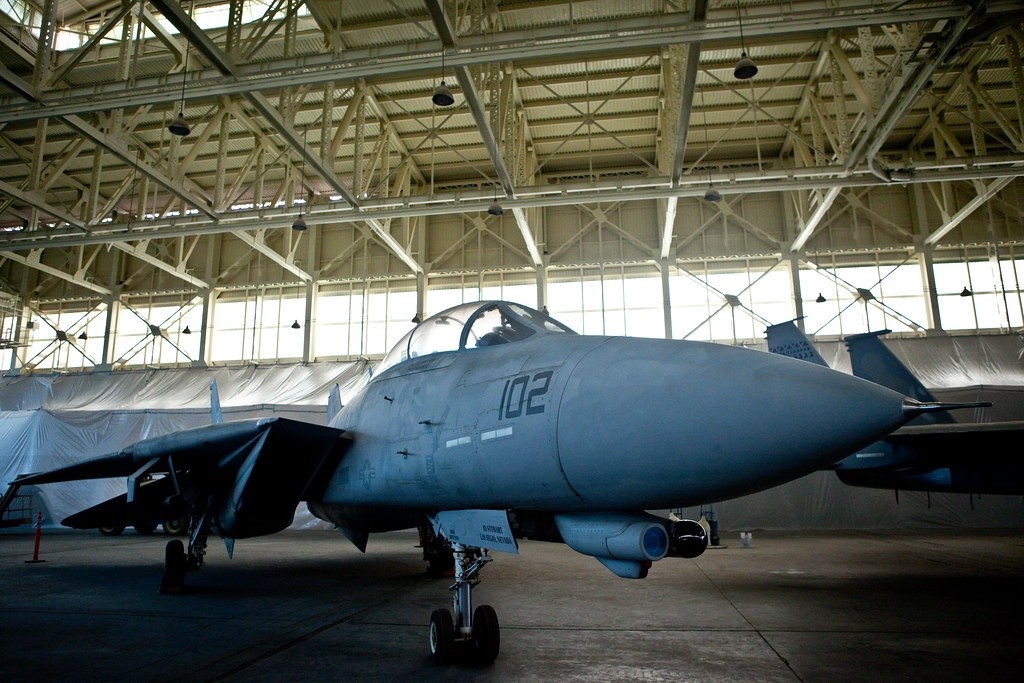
816, 293, 826, 302
292, 125, 307, 232
412, 254, 421, 323
957, 241, 973, 297
734, 0, 758, 80
432, 0, 455, 106
78, 331, 87, 340
168, 0, 194, 135
182, 274, 193, 334
291, 263, 300, 328
700, 84, 720, 201
488, 172, 504, 216
541, 305, 549, 316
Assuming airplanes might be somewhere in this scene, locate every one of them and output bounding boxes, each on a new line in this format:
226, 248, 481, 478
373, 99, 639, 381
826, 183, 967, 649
0, 296, 1023, 665
